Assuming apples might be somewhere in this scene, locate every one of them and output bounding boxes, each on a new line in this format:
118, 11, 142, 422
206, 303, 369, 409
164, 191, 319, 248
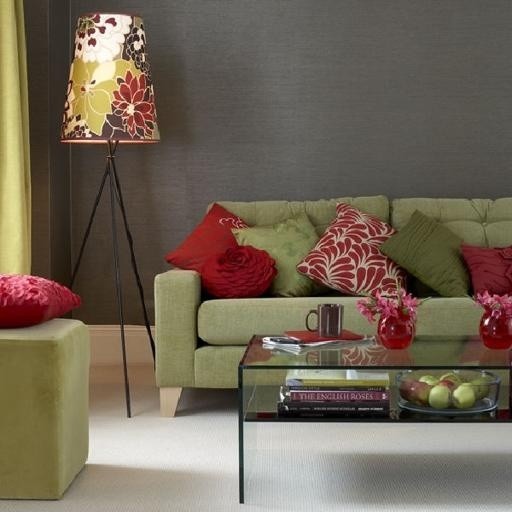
438, 381, 455, 391
454, 384, 476, 410
408, 383, 432, 406
399, 379, 416, 401
419, 375, 439, 385
441, 374, 461, 385
429, 385, 453, 409
470, 378, 491, 401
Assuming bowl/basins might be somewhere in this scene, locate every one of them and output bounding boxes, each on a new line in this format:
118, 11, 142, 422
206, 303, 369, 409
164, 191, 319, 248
395, 369, 501, 413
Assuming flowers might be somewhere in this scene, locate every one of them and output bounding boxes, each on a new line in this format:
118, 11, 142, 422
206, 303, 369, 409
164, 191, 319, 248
352, 277, 426, 334
466, 290, 512, 320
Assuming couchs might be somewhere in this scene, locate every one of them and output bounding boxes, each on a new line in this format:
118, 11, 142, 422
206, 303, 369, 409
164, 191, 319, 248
153, 194, 512, 424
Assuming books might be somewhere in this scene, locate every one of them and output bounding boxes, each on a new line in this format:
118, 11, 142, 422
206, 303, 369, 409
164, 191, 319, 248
276, 369, 389, 423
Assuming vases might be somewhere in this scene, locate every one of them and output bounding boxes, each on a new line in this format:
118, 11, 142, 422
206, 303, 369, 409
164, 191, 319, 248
479, 312, 512, 352
376, 317, 415, 350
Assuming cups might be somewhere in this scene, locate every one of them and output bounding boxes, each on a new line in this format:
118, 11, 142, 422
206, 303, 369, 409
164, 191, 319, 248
306, 304, 343, 338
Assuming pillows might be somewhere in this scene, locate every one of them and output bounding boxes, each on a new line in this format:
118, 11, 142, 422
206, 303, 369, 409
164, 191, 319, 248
378, 209, 472, 298
229, 212, 328, 298
165, 203, 277, 299
296, 202, 409, 298
0, 272, 83, 330
459, 242, 512, 299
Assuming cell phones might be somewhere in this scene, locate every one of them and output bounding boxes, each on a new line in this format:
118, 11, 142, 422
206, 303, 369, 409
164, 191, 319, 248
269, 337, 296, 345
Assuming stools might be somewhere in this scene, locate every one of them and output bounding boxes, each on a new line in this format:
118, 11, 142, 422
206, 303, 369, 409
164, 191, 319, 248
0, 317, 90, 501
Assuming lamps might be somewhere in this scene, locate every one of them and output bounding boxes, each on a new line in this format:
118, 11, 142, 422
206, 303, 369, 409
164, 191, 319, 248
59, 13, 161, 419
